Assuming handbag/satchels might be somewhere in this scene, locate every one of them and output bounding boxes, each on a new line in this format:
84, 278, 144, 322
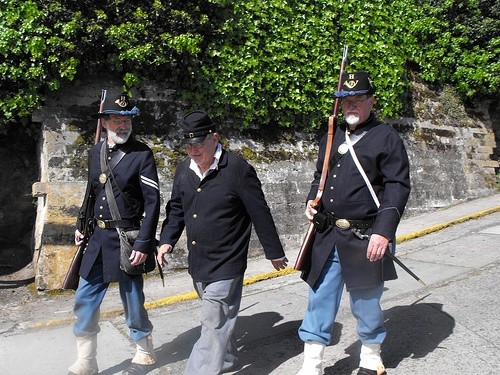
119, 230, 156, 276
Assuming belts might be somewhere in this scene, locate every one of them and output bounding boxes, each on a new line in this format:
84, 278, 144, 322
92, 215, 143, 231
321, 210, 375, 230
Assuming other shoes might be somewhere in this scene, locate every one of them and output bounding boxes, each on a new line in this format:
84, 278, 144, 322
357, 365, 388, 375
122, 359, 159, 375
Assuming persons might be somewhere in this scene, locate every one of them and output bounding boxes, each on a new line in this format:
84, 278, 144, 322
298, 72, 412, 375
157, 110, 289, 375
68, 92, 161, 375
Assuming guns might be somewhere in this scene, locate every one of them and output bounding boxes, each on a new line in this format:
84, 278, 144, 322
293, 44, 350, 272
60, 89, 107, 292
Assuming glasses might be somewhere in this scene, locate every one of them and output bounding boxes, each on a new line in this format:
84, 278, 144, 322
113, 118, 132, 125
341, 99, 363, 107
183, 141, 205, 150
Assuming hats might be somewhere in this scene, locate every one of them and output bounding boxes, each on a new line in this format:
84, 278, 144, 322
179, 109, 217, 146
334, 71, 377, 97
91, 91, 141, 119
